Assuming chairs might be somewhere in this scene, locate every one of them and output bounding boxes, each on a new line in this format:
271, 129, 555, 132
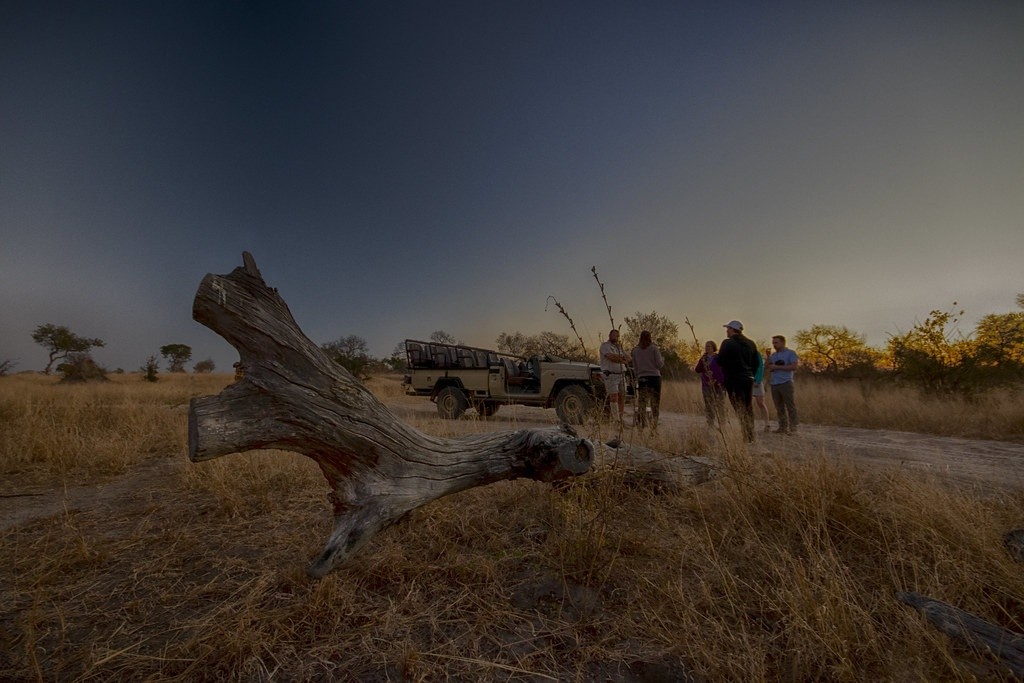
410, 343, 535, 386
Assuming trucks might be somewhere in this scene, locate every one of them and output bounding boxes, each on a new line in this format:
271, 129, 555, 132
402, 336, 637, 427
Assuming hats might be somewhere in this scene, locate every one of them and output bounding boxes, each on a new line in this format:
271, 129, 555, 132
723, 320, 744, 332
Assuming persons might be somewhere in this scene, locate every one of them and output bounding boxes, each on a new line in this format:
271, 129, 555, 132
696, 341, 727, 435
600, 330, 633, 433
632, 332, 664, 438
717, 320, 798, 444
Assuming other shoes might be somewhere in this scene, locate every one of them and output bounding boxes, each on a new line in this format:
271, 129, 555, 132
743, 438, 756, 446
771, 428, 788, 435
